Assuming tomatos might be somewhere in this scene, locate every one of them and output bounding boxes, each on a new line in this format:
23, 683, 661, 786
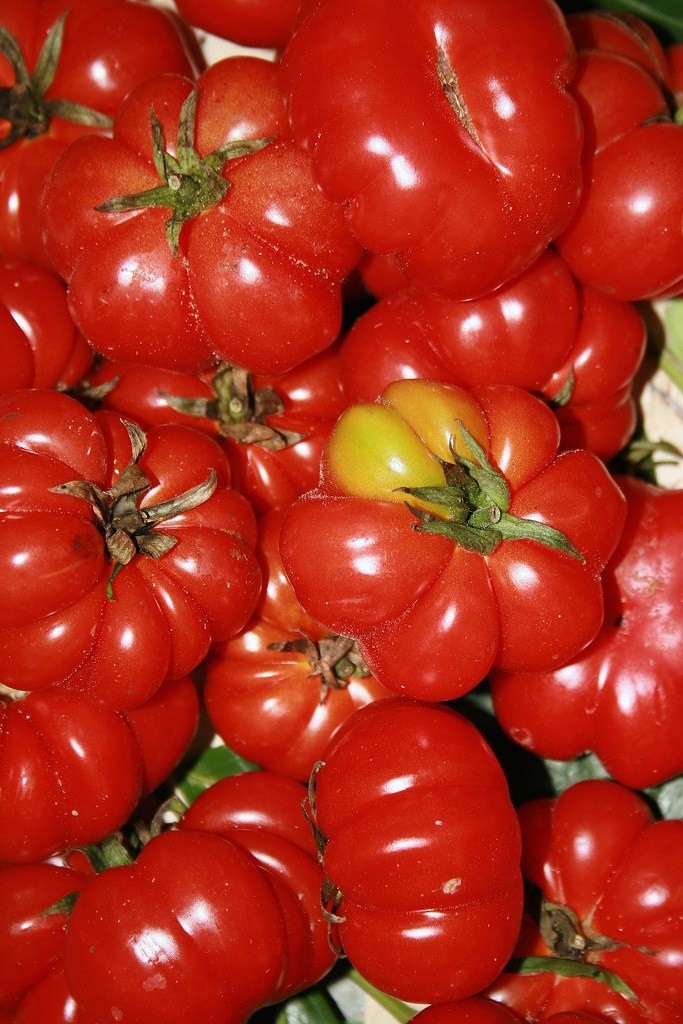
0, 0, 683, 1024
